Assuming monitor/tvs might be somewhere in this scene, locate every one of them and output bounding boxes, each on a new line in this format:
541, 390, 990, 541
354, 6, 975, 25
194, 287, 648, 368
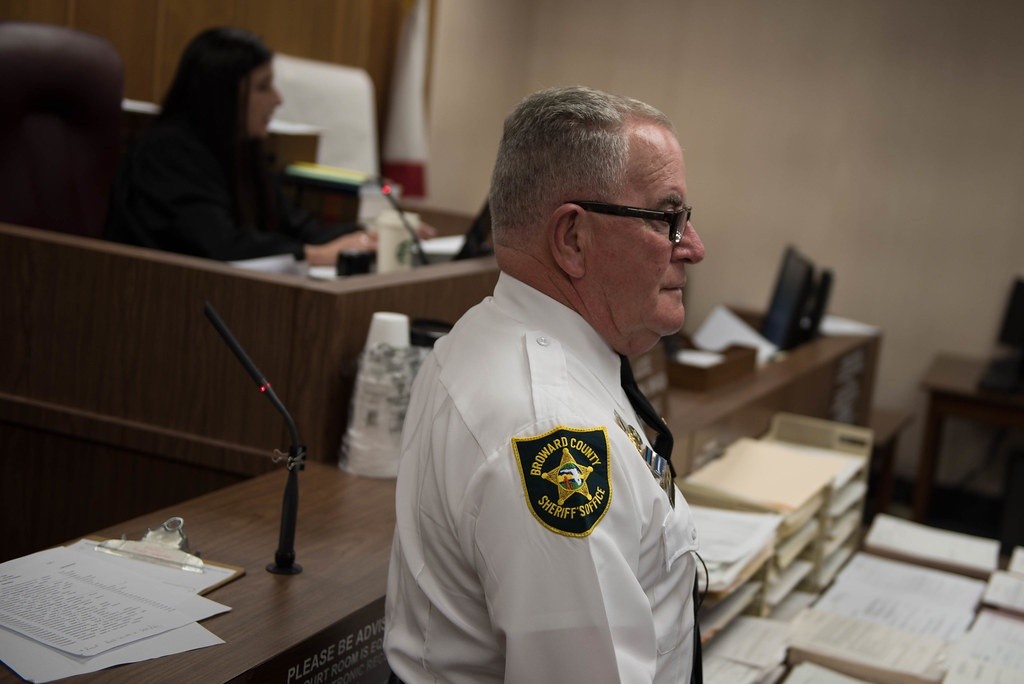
453, 200, 495, 261
761, 246, 833, 351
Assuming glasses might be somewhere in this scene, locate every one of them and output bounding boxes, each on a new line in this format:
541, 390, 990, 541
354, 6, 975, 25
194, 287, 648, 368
561, 200, 692, 245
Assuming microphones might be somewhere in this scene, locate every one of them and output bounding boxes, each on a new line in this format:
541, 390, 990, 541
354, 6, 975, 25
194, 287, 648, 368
198, 300, 305, 577
376, 176, 432, 268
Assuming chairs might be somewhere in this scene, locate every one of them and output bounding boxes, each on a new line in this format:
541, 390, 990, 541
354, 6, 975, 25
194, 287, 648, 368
915, 279, 1024, 544
0, 23, 124, 234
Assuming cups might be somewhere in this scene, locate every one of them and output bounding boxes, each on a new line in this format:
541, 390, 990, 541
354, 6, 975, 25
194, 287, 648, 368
376, 210, 419, 274
340, 312, 412, 480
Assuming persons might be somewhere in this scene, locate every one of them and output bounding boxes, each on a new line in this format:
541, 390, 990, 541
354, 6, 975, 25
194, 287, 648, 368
104, 27, 434, 267
382, 84, 705, 684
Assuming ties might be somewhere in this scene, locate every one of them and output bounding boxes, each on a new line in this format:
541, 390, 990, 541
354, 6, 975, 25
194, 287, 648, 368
616, 352, 676, 478
